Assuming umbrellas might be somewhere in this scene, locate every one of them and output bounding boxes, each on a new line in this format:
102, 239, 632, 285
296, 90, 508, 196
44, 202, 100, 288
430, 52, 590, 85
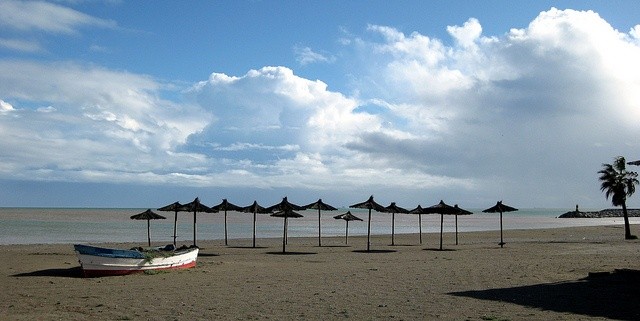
301, 199, 339, 211
172, 197, 218, 213
238, 201, 272, 214
376, 201, 409, 214
210, 199, 243, 211
348, 195, 386, 211
266, 196, 307, 211
130, 209, 168, 220
270, 210, 306, 218
333, 210, 364, 221
158, 201, 182, 211
481, 200, 519, 213
453, 204, 474, 215
407, 205, 424, 214
425, 200, 454, 214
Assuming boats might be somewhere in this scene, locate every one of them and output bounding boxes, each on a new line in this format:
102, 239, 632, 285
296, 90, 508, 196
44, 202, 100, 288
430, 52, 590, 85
73, 245, 198, 274
